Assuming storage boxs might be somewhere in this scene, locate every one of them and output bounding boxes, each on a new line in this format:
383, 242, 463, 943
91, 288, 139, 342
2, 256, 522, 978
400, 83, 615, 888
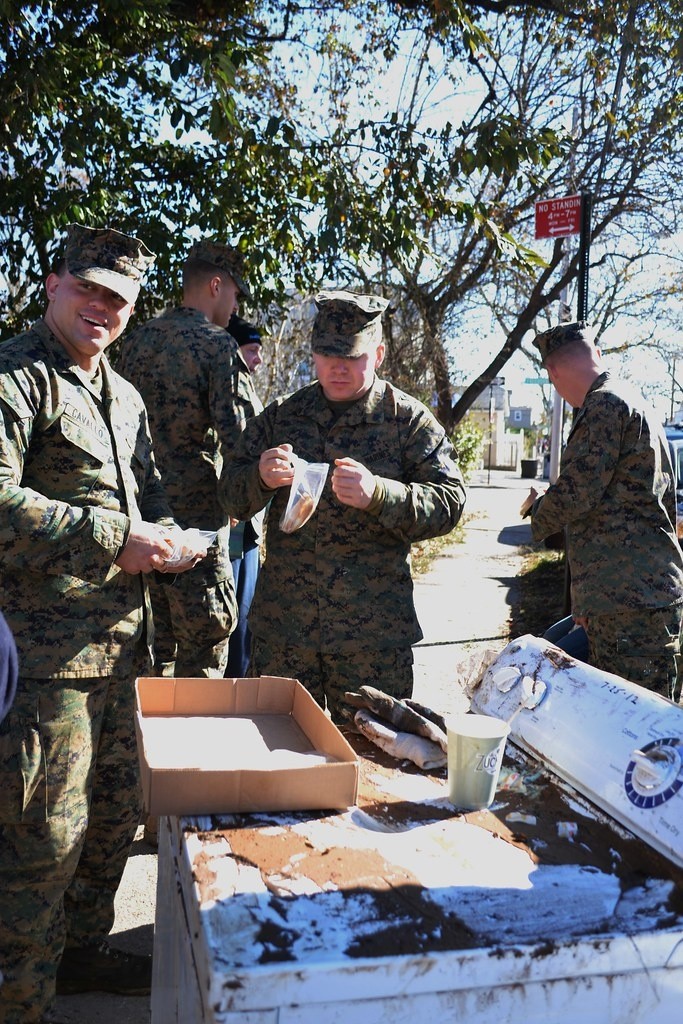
134, 674, 361, 815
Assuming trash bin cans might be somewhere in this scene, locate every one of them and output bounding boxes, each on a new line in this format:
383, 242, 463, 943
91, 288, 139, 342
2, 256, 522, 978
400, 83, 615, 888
520, 459, 539, 479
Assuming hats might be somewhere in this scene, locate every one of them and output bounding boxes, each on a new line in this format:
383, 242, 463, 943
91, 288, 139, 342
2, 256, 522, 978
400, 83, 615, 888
532, 320, 589, 368
313, 288, 393, 360
55, 223, 158, 305
227, 315, 261, 346
189, 240, 252, 297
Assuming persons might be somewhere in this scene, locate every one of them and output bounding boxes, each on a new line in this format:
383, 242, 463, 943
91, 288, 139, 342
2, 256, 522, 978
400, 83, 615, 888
0, 221, 208, 1024
544, 614, 589, 654
224, 316, 266, 680
215, 291, 467, 720
528, 319, 683, 703
114, 237, 264, 678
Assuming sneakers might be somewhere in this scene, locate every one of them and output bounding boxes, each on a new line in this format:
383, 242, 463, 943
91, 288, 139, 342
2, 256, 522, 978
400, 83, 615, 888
57, 942, 152, 994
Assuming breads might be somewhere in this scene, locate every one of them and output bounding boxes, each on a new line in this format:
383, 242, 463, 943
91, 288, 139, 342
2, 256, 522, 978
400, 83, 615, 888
284, 495, 313, 533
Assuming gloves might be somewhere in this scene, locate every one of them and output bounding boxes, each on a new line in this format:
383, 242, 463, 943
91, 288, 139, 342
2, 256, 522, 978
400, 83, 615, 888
335, 682, 452, 771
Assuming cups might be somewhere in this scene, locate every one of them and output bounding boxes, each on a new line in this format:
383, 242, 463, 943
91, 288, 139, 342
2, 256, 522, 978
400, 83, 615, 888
443, 713, 512, 810
